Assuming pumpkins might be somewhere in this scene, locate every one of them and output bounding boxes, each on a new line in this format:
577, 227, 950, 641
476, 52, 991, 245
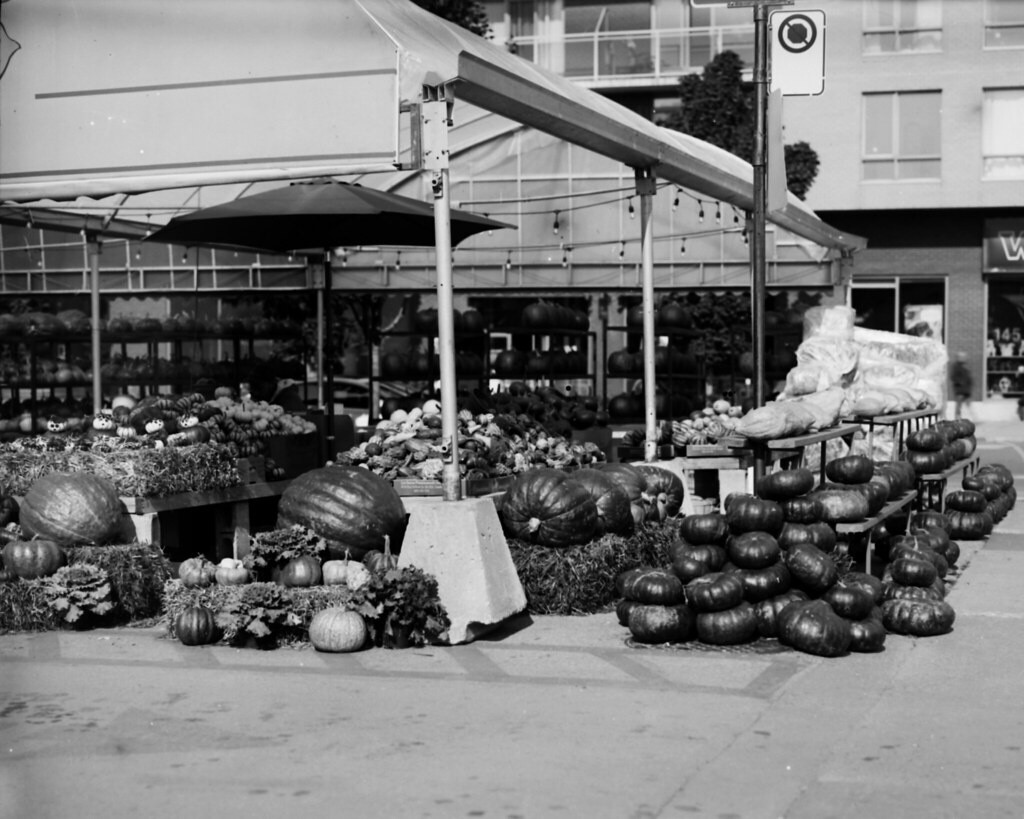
616, 399, 1018, 657
0, 308, 318, 450
498, 462, 684, 548
0, 468, 122, 580
172, 465, 410, 653
328, 297, 670, 477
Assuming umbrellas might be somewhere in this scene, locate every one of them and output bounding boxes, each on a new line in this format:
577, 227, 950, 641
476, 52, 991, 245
140, 176, 518, 409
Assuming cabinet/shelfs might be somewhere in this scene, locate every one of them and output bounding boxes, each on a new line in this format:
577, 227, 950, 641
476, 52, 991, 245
0, 317, 598, 430
602, 316, 804, 424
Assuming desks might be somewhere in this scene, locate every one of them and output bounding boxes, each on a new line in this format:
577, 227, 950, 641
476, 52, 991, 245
11, 478, 295, 560
839, 405, 939, 461
832, 490, 920, 578
401, 491, 506, 516
726, 423, 860, 496
919, 455, 980, 516
